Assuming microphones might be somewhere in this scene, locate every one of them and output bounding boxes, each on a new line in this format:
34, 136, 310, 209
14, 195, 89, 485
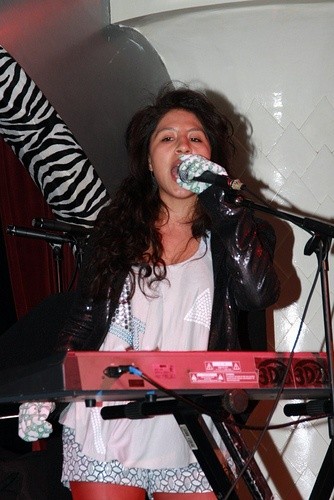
176, 161, 244, 194
5, 216, 95, 249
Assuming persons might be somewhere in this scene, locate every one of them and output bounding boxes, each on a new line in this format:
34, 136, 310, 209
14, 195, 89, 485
18, 83, 279, 498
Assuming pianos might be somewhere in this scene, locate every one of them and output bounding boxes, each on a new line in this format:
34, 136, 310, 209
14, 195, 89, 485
1, 351, 333, 403
